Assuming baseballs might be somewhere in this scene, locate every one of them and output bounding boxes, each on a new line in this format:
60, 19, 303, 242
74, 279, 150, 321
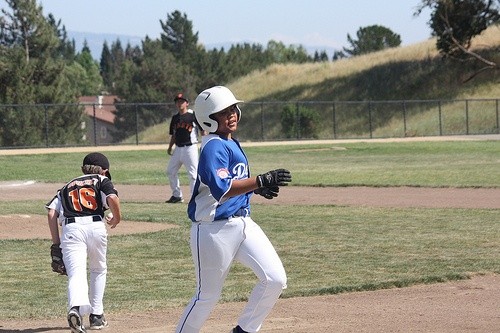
107, 212, 114, 220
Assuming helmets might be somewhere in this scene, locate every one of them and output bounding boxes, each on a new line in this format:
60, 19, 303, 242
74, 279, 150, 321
194, 86, 242, 132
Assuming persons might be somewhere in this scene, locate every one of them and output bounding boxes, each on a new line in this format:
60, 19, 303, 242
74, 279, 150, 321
45, 153, 121, 333
176, 86, 292, 333
166, 93, 206, 203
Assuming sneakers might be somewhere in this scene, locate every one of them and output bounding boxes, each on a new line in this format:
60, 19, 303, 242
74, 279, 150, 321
67, 308, 87, 333
89, 314, 109, 329
165, 196, 184, 203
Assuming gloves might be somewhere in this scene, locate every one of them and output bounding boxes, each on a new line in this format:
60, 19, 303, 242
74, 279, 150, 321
258, 169, 292, 188
255, 185, 280, 199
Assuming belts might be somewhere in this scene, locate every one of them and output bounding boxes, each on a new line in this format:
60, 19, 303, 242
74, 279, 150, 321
65, 215, 101, 225
178, 143, 193, 147
234, 208, 251, 218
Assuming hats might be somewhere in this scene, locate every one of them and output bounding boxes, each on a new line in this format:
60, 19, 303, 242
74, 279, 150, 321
83, 152, 111, 181
173, 93, 189, 103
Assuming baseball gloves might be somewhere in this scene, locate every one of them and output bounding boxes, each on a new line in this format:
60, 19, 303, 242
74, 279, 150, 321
49, 243, 67, 276
